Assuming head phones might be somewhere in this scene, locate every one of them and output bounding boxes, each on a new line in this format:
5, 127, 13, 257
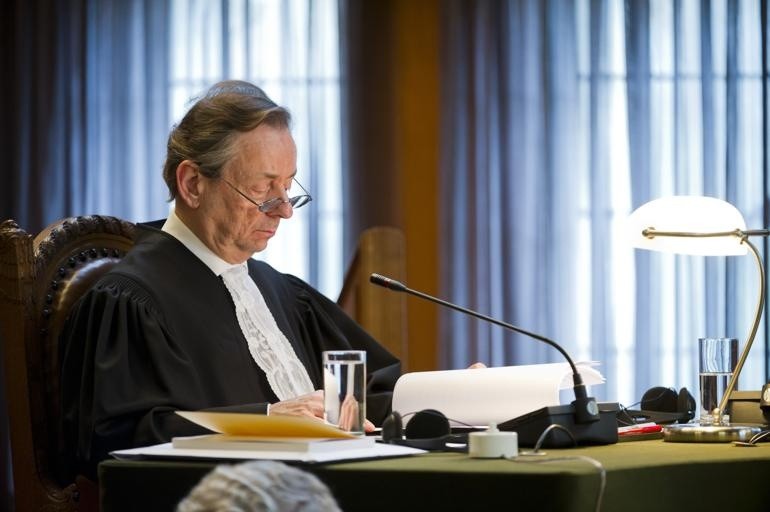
381, 404, 484, 452
639, 381, 698, 423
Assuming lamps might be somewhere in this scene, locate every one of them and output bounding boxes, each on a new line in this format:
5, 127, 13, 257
616, 194, 766, 442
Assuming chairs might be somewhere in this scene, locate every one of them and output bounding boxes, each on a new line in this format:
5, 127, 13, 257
2, 212, 168, 511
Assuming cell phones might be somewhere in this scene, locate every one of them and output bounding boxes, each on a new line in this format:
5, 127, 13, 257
662, 419, 763, 445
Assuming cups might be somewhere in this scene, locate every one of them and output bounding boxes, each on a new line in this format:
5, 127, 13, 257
322, 349, 366, 436
699, 338, 738, 428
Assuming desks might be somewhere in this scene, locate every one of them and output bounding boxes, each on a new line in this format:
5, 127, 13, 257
92, 412, 770, 512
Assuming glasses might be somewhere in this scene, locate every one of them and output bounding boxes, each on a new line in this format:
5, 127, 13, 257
215, 178, 312, 213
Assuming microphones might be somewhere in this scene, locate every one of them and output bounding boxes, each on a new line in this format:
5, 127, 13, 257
368, 270, 622, 450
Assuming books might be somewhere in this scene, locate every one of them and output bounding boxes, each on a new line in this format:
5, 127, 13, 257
171, 410, 376, 453
370, 358, 606, 433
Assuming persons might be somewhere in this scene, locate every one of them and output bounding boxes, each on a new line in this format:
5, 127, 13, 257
49, 79, 487, 487
